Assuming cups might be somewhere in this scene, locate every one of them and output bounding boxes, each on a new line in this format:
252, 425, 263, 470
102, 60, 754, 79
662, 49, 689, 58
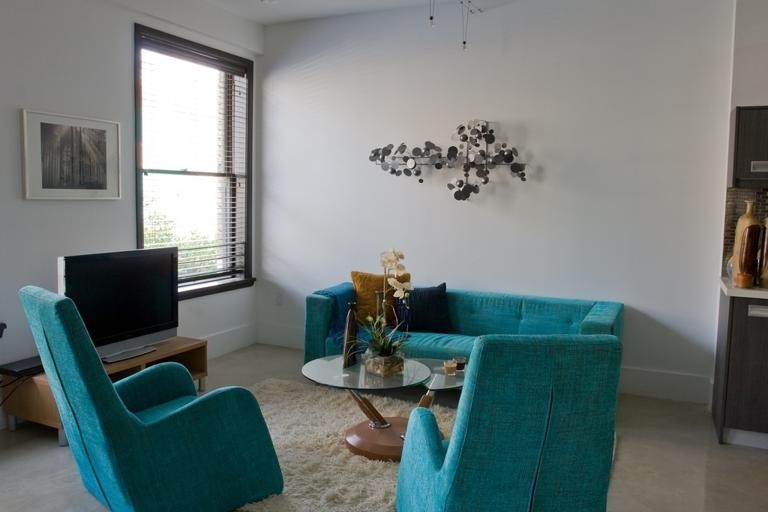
444, 360, 457, 375
453, 357, 466, 370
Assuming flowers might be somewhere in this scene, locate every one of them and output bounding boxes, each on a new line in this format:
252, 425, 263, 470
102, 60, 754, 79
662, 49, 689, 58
344, 251, 412, 355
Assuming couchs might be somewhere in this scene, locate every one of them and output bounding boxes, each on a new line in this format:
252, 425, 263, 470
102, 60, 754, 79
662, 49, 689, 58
303, 282, 624, 396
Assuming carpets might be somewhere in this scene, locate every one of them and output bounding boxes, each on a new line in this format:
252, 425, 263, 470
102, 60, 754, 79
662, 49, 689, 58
236, 378, 618, 512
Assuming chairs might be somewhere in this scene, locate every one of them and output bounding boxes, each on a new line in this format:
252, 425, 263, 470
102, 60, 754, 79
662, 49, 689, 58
395, 333, 624, 512
19, 285, 284, 512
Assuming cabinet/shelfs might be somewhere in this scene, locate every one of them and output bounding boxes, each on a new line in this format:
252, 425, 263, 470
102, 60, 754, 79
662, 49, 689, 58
726, 104, 768, 187
711, 289, 768, 450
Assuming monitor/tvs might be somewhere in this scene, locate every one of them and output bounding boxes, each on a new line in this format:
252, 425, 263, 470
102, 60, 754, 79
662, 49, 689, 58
57, 246, 178, 363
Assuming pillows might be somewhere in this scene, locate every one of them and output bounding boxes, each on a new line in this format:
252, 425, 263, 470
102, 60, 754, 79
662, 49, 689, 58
350, 271, 410, 329
394, 283, 453, 333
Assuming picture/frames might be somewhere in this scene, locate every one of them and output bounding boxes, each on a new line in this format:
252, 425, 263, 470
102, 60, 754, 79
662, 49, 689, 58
22, 109, 122, 200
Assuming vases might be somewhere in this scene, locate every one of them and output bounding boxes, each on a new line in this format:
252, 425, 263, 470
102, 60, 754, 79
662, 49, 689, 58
360, 352, 404, 378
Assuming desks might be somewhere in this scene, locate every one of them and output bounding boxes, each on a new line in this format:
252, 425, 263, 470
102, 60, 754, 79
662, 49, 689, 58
6, 334, 208, 446
